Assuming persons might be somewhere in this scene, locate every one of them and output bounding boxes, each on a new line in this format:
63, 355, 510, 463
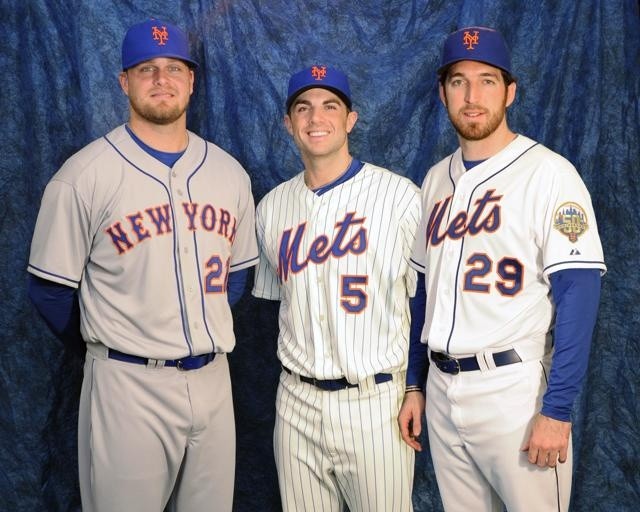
396, 26, 609, 512
26, 16, 260, 512
250, 65, 422, 512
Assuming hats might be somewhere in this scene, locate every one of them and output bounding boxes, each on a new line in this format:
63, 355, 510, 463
122, 19, 200, 72
285, 65, 352, 104
436, 27, 514, 77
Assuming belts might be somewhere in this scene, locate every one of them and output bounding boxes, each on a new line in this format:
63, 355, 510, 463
430, 349, 523, 375
282, 366, 393, 392
108, 347, 216, 373
